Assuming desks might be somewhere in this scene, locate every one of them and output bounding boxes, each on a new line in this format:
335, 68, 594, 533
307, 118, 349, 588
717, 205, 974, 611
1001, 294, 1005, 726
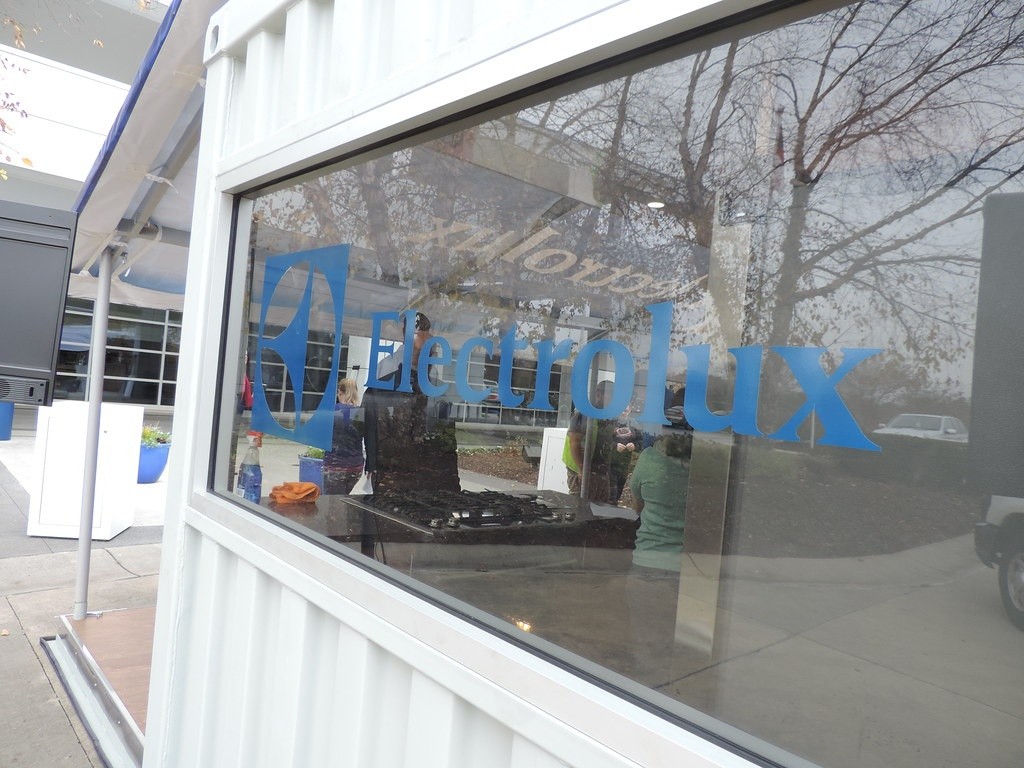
258, 488, 642, 561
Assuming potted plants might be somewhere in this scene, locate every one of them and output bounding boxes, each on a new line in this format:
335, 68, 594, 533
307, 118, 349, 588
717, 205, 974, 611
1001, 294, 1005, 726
137, 420, 172, 483
298, 446, 325, 495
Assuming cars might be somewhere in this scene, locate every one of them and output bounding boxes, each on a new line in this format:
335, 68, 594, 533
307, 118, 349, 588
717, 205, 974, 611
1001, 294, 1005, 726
871, 410, 966, 441
662, 404, 694, 430
973, 491, 1024, 634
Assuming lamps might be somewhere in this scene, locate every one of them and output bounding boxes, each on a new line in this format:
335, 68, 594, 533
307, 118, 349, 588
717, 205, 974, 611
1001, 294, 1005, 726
522, 444, 542, 469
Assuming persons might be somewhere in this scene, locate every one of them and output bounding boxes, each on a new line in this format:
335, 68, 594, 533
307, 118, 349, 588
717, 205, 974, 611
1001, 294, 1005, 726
320, 372, 364, 496
630, 407, 693, 693
364, 312, 463, 496
562, 379, 644, 508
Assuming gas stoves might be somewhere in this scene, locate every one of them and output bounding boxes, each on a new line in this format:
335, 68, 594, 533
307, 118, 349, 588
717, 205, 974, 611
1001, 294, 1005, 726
364, 488, 553, 528
